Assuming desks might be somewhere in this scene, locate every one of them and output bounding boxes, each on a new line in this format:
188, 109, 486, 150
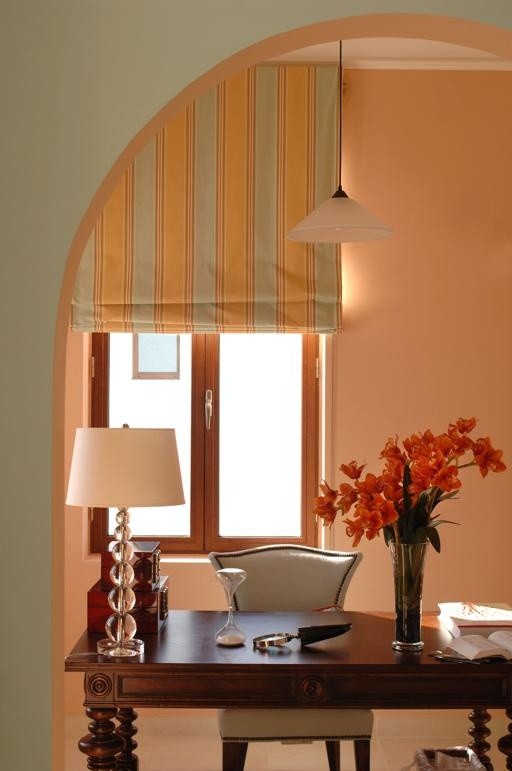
65, 610, 511, 771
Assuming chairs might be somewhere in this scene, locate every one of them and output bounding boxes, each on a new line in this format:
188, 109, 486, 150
208, 547, 375, 771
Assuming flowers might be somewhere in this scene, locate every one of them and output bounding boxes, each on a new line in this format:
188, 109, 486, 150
314, 417, 505, 642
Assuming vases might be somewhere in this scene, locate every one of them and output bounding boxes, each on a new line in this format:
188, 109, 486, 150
387, 539, 430, 651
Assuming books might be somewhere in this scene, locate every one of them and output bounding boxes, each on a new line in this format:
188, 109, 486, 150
437, 601, 512, 661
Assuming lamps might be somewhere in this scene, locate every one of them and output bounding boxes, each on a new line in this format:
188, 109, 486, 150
65, 422, 187, 660
287, 39, 393, 246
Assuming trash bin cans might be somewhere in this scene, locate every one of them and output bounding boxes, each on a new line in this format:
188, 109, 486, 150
416, 745, 486, 771
